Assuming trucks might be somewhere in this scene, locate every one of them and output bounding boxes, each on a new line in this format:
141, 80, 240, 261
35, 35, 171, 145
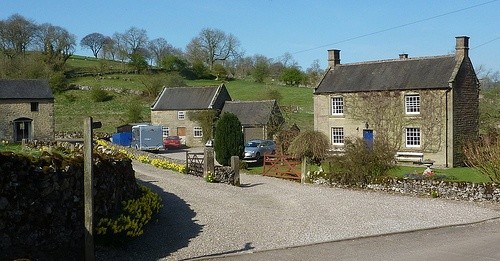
130, 126, 164, 154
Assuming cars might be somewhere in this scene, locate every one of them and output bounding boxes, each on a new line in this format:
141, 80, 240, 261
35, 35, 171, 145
243, 139, 277, 162
163, 135, 182, 150
